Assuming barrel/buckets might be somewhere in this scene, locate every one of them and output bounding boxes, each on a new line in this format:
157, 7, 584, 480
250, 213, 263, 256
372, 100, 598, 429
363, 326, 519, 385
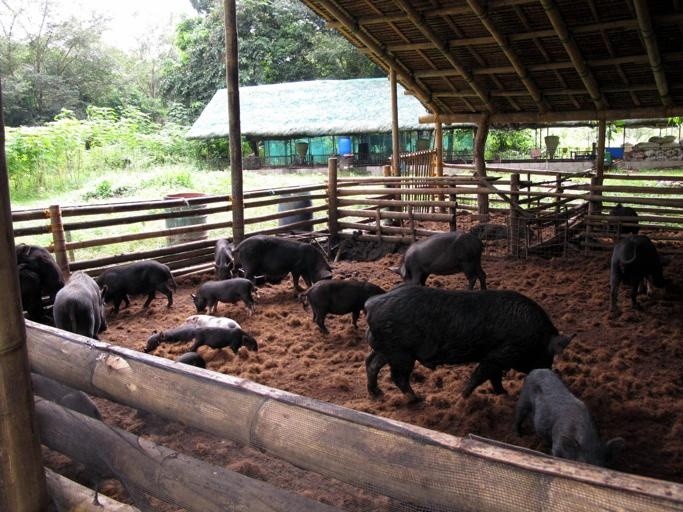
278, 188, 313, 230
337, 136, 352, 154
166, 192, 208, 245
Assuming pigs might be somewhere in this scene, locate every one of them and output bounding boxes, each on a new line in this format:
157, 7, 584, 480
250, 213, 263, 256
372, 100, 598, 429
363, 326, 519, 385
609, 234, 672, 308
609, 203, 641, 232
468, 221, 539, 241
14, 243, 177, 338
388, 231, 487, 291
144, 314, 259, 352
298, 279, 387, 333
215, 236, 339, 290
514, 368, 627, 467
363, 287, 578, 405
177, 352, 207, 369
190, 276, 260, 317
